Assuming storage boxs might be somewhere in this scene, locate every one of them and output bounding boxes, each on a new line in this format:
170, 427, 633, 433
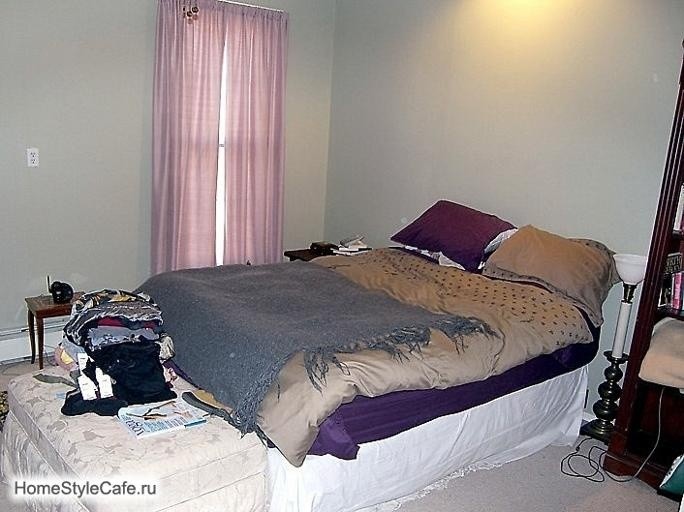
0, 365, 272, 512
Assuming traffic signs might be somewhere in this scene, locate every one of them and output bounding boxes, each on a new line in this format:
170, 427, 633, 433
27, 147, 40, 168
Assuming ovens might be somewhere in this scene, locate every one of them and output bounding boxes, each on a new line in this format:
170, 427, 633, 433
128, 246, 601, 512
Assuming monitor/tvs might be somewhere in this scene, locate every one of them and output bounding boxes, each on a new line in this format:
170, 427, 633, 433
657, 252, 684, 310
671, 184, 684, 232
117, 398, 207, 440
330, 236, 372, 256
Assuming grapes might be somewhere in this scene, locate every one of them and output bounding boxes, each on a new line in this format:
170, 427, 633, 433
284, 249, 373, 264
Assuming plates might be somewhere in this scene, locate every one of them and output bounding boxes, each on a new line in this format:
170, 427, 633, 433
603, 39, 684, 502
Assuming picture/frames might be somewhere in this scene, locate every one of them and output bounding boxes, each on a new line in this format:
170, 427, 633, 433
25, 292, 89, 369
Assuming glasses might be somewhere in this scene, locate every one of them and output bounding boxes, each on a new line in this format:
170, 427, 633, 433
580, 254, 649, 444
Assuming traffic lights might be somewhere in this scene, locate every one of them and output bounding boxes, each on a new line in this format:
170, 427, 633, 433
391, 198, 622, 327
